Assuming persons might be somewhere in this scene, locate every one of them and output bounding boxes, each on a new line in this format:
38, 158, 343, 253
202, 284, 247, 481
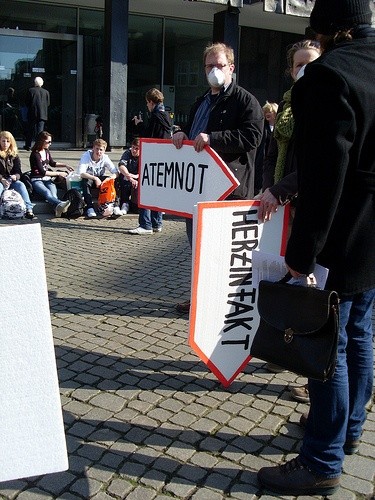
255, 0, 374, 493
256, 41, 322, 401
0, 131, 37, 219
24, 76, 50, 150
77, 138, 140, 218
29, 132, 74, 217
1, 88, 18, 133
127, 89, 181, 235
172, 43, 264, 311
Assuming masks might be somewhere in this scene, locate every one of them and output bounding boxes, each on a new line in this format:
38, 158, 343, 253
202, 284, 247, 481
207, 67, 226, 87
295, 65, 306, 82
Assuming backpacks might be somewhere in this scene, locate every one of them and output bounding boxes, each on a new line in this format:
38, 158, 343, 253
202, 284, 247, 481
0, 189, 26, 220
61, 189, 85, 220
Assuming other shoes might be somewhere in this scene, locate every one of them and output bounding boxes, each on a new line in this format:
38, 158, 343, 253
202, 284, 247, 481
267, 362, 290, 373
23, 145, 30, 151
113, 206, 123, 216
127, 227, 153, 235
120, 203, 129, 215
56, 200, 70, 217
87, 208, 97, 217
300, 412, 360, 455
290, 384, 310, 402
257, 456, 341, 495
152, 227, 162, 232
26, 211, 34, 219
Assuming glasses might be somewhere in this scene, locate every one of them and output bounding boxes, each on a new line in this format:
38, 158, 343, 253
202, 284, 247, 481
204, 63, 231, 69
289, 63, 304, 69
44, 140, 52, 143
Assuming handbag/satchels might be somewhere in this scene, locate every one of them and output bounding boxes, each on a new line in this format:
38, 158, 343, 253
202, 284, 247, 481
98, 179, 116, 206
250, 269, 339, 382
